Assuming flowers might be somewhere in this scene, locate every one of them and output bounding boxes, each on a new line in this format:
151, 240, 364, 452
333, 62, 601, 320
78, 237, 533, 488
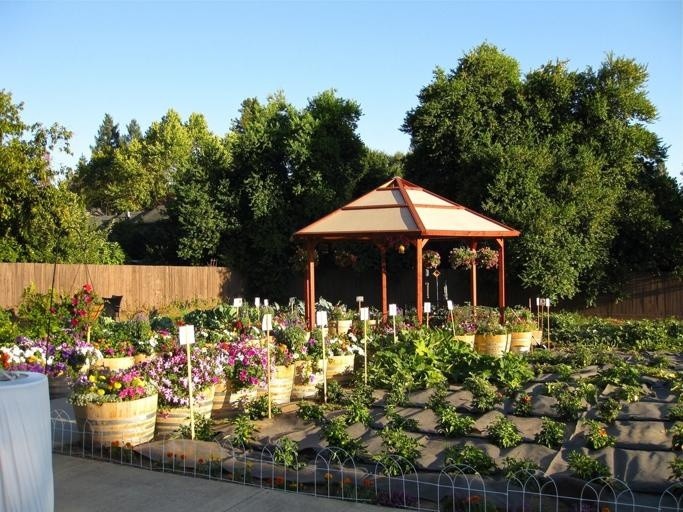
422, 245, 500, 271
